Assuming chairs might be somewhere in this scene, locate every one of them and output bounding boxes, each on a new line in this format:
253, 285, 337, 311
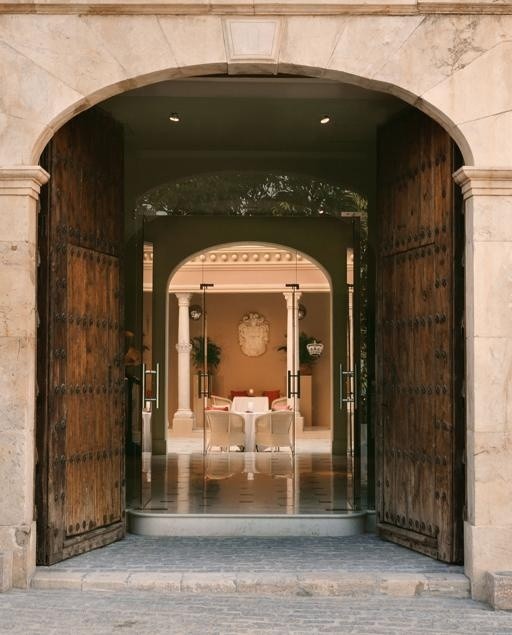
204, 390, 295, 452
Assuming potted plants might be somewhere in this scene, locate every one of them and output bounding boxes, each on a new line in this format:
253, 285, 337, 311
190, 335, 222, 375
278, 331, 322, 375
